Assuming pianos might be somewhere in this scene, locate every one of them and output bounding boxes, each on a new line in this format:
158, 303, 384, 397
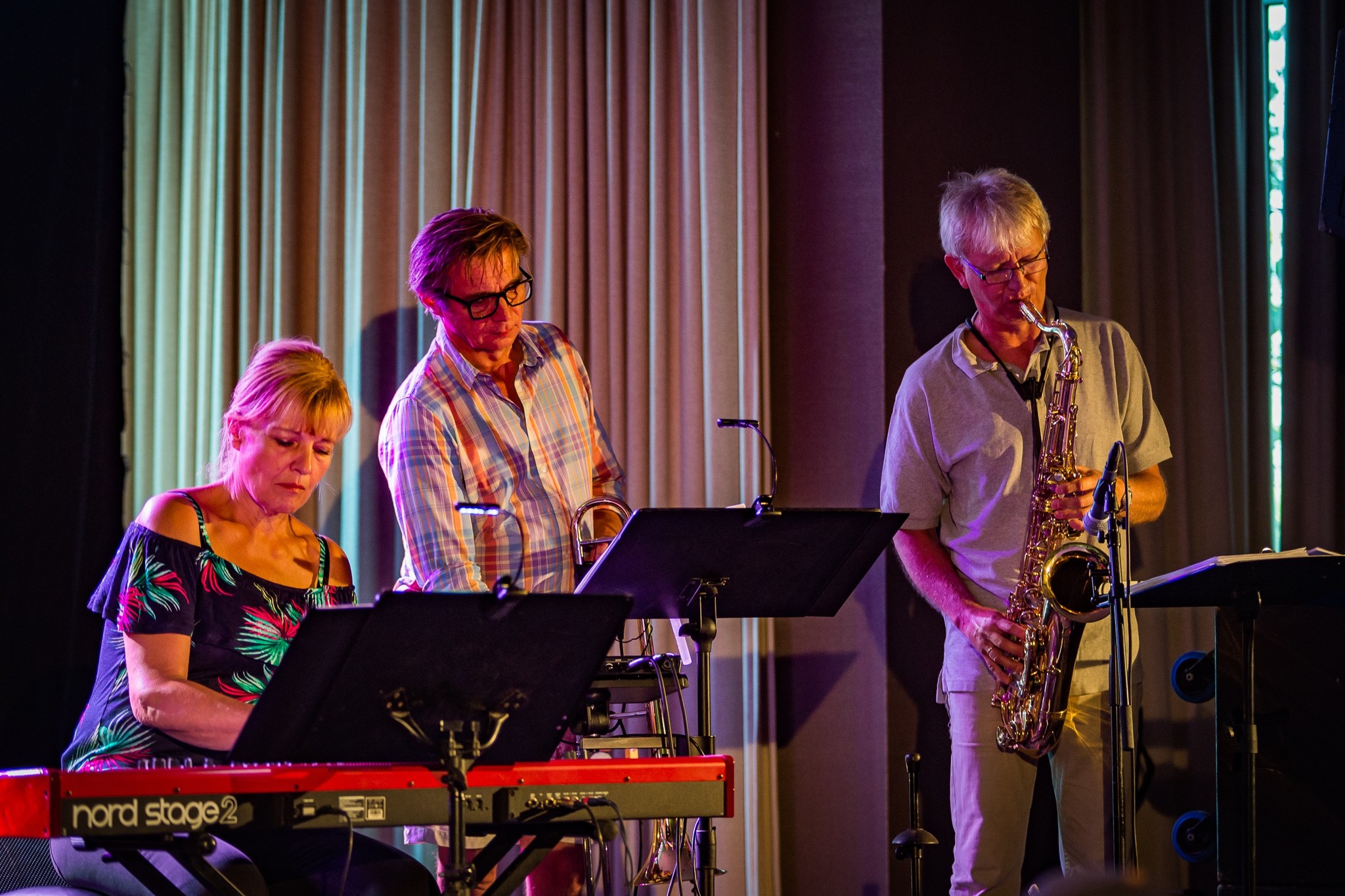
0, 755, 735, 896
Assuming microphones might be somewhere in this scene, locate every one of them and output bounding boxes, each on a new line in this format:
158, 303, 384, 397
1081, 441, 1123, 537
717, 419, 778, 507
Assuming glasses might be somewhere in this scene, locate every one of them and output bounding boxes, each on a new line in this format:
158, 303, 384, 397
441, 264, 533, 320
960, 237, 1049, 285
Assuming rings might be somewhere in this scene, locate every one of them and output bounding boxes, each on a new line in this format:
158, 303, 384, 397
987, 646, 994, 655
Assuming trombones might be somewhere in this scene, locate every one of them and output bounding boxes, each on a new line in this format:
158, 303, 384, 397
568, 493, 729, 896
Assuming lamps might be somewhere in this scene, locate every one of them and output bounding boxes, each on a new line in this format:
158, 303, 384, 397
455, 500, 528, 592
717, 417, 781, 517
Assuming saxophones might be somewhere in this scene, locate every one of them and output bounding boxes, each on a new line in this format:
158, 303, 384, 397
990, 301, 1115, 757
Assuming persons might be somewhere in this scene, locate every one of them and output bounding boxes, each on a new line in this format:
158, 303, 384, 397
374, 207, 631, 895
47, 340, 441, 896
878, 167, 1172, 896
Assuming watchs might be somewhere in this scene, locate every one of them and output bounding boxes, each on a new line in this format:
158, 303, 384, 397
1117, 474, 1133, 514
